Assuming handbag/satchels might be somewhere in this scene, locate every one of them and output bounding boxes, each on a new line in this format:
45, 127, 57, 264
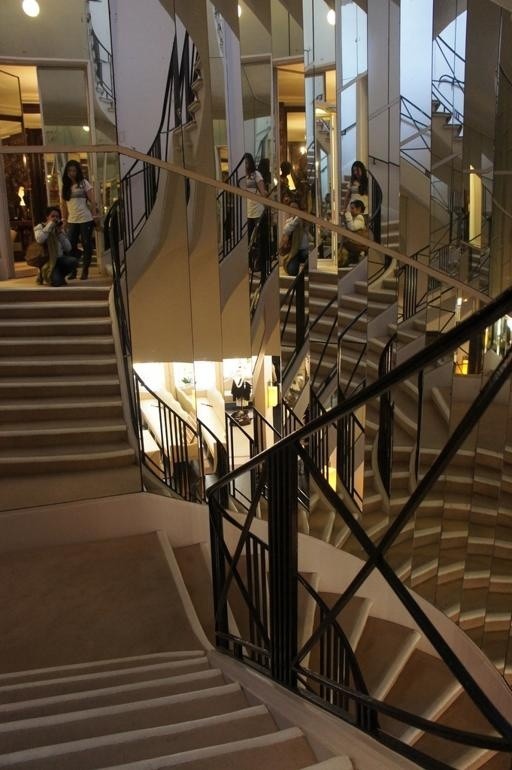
27, 242, 49, 266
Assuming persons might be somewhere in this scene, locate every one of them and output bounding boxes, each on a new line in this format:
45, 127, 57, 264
58, 160, 105, 282
240, 154, 384, 276
23, 206, 79, 286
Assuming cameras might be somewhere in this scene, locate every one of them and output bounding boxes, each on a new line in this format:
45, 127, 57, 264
55, 219, 63, 226
